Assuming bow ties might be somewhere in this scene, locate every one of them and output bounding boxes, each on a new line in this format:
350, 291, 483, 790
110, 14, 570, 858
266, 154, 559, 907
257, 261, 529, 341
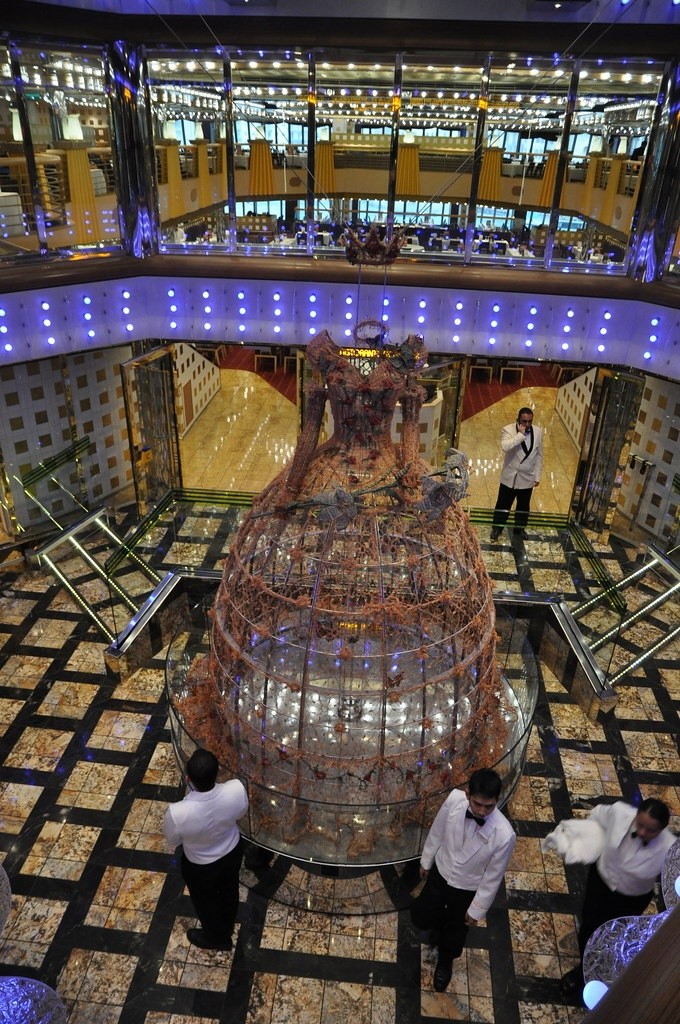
525, 430, 531, 434
631, 831, 649, 847
465, 810, 485, 826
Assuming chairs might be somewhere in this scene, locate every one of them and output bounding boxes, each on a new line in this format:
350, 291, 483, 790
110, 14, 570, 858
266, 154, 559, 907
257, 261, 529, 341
432, 240, 442, 251
297, 234, 307, 246
495, 243, 506, 255
408, 238, 412, 244
479, 242, 489, 254
526, 163, 544, 179
314, 235, 324, 246
449, 240, 460, 251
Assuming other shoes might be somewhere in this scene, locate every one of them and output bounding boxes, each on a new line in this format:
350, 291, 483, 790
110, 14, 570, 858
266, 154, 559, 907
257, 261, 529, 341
490, 529, 500, 540
187, 927, 233, 951
562, 966, 583, 990
513, 528, 529, 540
434, 956, 453, 992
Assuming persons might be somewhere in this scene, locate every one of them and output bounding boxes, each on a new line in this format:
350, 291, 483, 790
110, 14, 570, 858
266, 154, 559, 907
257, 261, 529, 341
489, 407, 542, 540
561, 795, 678, 992
408, 767, 517, 993
162, 747, 249, 950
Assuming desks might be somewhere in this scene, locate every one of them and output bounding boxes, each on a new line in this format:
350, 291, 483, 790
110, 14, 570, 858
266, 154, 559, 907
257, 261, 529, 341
295, 232, 333, 245
408, 236, 419, 245
568, 165, 587, 183
502, 163, 545, 178
234, 153, 250, 169
473, 240, 509, 255
269, 238, 295, 246
602, 172, 638, 191
571, 246, 582, 260
286, 153, 308, 168
429, 238, 464, 250
507, 249, 535, 258
